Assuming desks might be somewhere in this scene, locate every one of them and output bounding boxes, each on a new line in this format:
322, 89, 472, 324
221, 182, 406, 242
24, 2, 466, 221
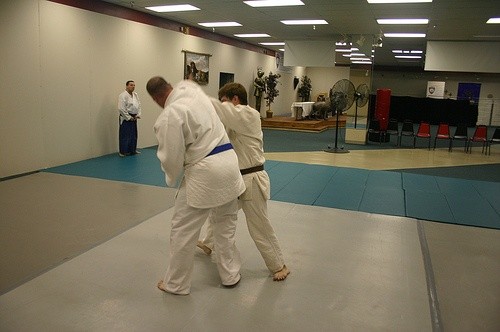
291, 101, 316, 119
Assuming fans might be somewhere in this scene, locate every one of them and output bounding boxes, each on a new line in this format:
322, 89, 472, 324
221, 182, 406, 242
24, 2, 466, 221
354, 84, 369, 128
323, 79, 356, 153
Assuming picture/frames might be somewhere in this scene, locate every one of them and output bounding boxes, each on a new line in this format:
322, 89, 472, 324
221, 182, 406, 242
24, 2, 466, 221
184, 51, 210, 86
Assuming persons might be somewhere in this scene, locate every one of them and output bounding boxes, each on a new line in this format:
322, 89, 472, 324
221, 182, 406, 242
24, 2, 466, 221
253, 69, 266, 114
144, 76, 243, 294
192, 82, 290, 281
116, 81, 142, 157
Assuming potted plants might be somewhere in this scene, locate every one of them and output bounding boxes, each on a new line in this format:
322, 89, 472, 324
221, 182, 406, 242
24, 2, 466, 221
265, 72, 281, 118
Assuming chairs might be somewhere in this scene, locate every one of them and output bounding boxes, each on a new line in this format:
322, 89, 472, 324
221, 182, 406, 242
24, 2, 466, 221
366, 120, 430, 151
467, 126, 488, 155
489, 128, 500, 156
449, 125, 468, 152
433, 123, 452, 152
